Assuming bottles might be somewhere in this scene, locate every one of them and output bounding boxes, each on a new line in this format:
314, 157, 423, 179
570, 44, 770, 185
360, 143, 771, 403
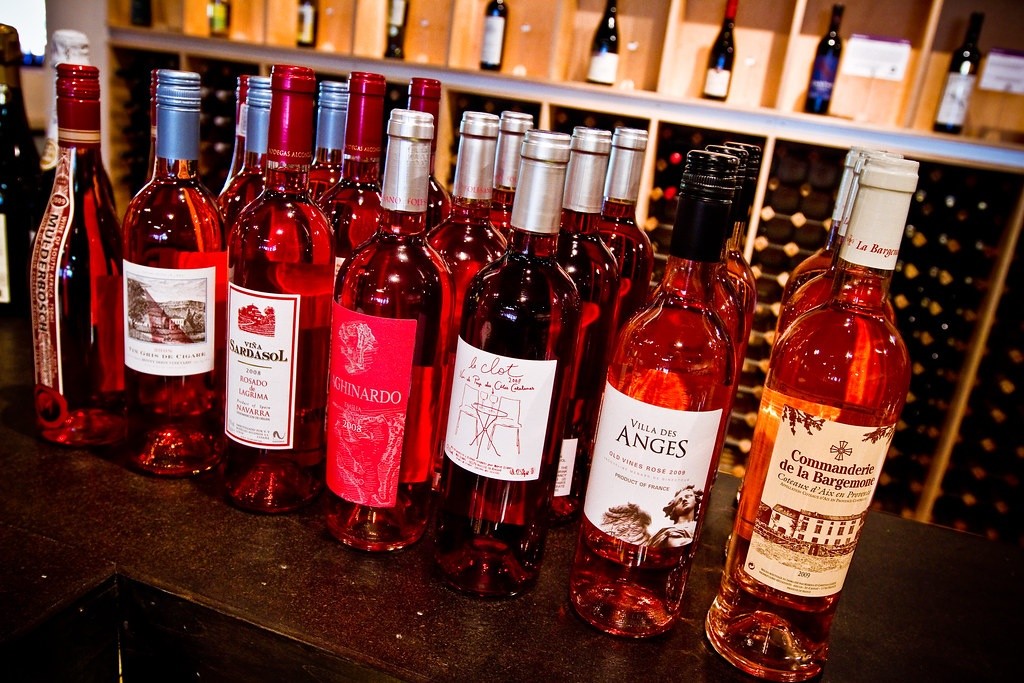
383, 0, 410, 59
585, 0, 620, 85
701, 0, 740, 102
0, 22, 1024, 682
208, 0, 231, 39
803, 3, 845, 114
931, 8, 985, 133
295, 0, 318, 48
130, 0, 152, 28
480, 0, 508, 72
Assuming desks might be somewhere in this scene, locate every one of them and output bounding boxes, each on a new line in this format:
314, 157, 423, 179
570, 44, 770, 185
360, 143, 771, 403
0, 309, 1024, 683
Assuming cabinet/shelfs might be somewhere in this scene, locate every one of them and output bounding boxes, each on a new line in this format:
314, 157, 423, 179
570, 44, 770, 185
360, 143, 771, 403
105, 0, 1024, 521
